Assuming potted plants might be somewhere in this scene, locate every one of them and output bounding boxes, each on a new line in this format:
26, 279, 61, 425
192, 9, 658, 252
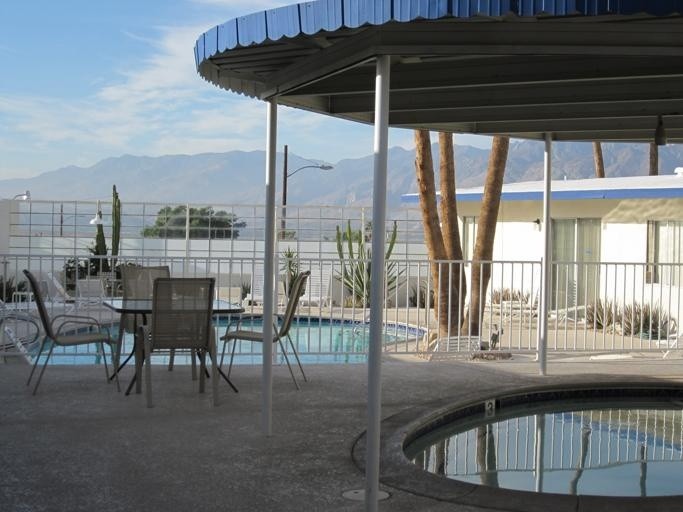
93, 182, 122, 283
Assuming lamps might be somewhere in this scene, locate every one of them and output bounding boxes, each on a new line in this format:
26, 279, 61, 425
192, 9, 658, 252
652, 111, 668, 147
89, 199, 106, 225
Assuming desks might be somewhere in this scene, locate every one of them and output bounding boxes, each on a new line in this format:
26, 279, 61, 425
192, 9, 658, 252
102, 299, 245, 394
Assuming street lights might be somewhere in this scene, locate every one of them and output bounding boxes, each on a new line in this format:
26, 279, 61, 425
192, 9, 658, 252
90, 198, 106, 226
281, 144, 335, 241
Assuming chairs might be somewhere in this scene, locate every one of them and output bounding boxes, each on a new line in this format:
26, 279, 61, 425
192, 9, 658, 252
216, 267, 314, 391
23, 267, 124, 396
119, 263, 199, 382
45, 271, 290, 304
135, 277, 217, 409
